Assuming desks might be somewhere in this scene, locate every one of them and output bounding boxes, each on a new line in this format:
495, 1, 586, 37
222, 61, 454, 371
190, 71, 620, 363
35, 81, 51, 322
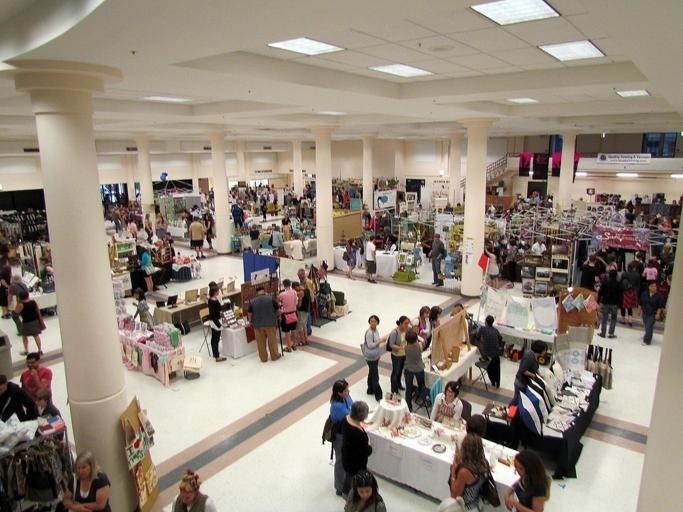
119, 286, 256, 386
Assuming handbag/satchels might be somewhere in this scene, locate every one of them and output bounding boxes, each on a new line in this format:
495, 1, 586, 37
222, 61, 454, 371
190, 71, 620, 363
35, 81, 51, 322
587, 345, 612, 389
0, 430, 72, 511
483, 477, 500, 506
386, 336, 392, 351
343, 252, 349, 260
306, 261, 344, 319
145, 264, 155, 276
38, 318, 46, 330
360, 344, 385, 361
323, 415, 336, 441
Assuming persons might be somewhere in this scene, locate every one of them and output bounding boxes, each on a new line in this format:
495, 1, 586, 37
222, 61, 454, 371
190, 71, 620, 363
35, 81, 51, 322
102, 180, 460, 362
329, 304, 551, 512
64, 451, 112, 511
485, 192, 683, 346
2, 256, 58, 416
171, 469, 216, 512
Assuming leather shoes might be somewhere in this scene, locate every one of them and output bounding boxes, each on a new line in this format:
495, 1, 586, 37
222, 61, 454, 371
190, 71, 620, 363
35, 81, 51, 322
368, 279, 376, 283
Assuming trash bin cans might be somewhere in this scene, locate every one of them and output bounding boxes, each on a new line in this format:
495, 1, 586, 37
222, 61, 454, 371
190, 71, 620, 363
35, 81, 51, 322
0, 328, 13, 381
230, 237, 241, 255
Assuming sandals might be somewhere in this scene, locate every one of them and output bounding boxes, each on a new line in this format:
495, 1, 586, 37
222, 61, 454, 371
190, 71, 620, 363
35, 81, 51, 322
283, 341, 308, 352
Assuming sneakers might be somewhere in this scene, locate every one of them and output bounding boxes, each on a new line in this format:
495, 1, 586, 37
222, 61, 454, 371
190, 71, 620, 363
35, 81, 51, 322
216, 358, 226, 361
619, 320, 632, 327
597, 333, 617, 338
432, 283, 443, 286
